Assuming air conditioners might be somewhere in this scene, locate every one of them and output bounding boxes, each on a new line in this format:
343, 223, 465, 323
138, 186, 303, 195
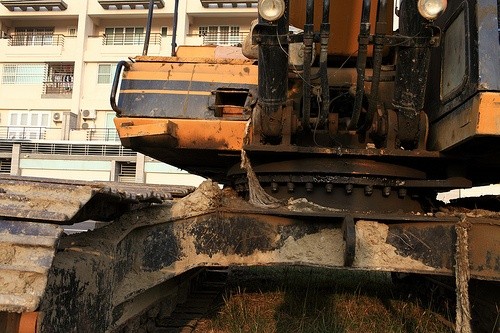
53, 112, 63, 122
82, 122, 96, 131
82, 109, 97, 120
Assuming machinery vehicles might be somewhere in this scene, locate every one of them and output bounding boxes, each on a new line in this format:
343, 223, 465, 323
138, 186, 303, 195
0, 0, 500, 333
